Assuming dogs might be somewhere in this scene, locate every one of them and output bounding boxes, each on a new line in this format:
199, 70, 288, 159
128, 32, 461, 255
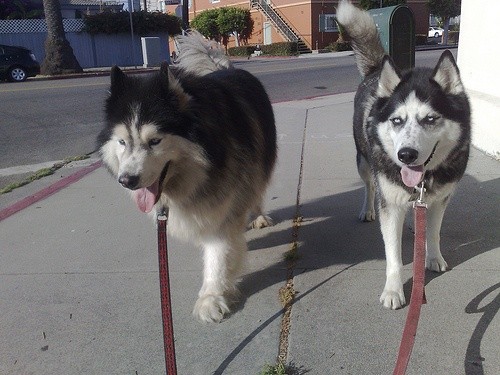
96, 28, 277, 325
335, 0, 472, 310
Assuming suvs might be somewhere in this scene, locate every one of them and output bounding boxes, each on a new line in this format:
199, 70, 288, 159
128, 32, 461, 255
0, 45, 40, 82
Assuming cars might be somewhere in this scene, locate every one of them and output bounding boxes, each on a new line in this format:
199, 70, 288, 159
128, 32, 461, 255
428, 26, 450, 38
